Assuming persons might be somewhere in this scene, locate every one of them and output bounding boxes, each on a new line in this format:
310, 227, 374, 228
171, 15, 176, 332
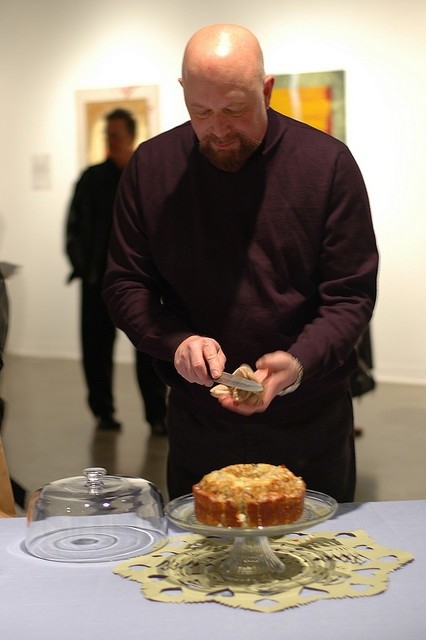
66, 106, 172, 437
107, 23, 381, 505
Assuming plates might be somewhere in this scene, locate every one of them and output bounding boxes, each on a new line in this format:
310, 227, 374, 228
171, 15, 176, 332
163, 489, 338, 578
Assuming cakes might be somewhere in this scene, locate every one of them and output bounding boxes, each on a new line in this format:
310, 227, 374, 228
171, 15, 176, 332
191, 458, 309, 531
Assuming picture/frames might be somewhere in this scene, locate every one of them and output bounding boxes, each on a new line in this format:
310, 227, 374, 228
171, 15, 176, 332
73, 83, 160, 166
265, 70, 346, 147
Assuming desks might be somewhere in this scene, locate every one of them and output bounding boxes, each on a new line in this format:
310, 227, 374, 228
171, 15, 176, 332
0, 500, 426, 640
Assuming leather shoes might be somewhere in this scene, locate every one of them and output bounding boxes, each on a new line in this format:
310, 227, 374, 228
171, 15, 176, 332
150, 420, 167, 436
96, 417, 121, 431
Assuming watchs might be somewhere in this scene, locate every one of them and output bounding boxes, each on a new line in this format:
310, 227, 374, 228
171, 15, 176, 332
275, 353, 305, 396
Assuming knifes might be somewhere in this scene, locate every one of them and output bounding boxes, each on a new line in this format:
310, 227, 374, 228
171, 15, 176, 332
206, 371, 263, 393
